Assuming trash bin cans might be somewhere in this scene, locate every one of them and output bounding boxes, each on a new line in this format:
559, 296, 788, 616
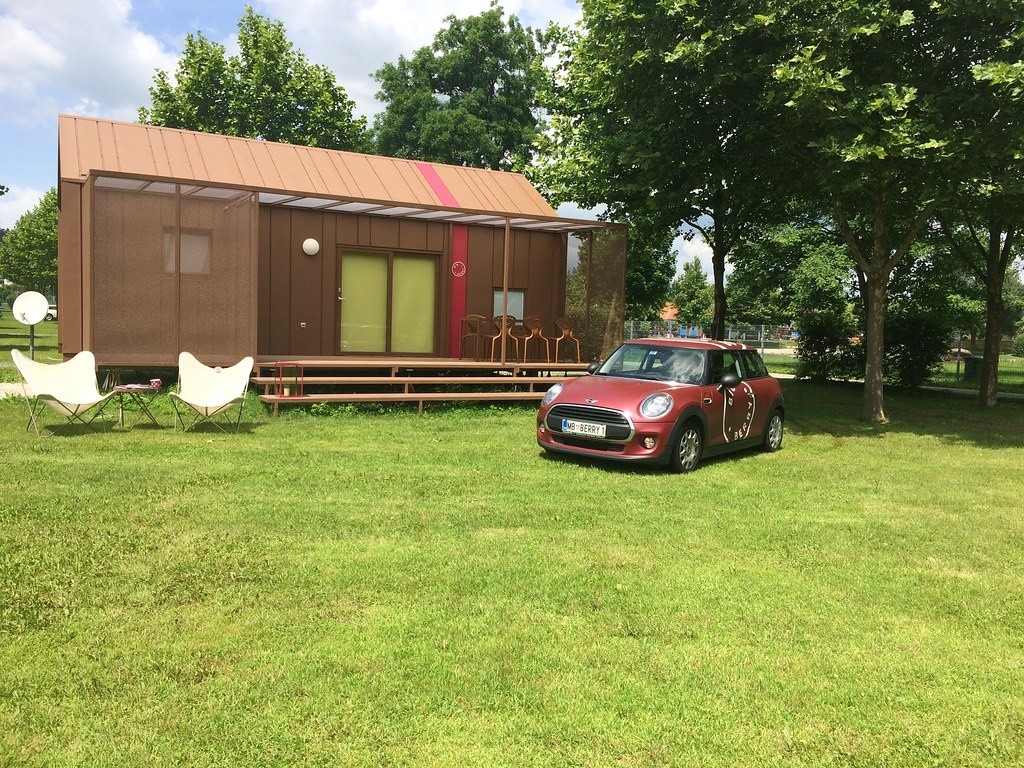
965, 356, 983, 381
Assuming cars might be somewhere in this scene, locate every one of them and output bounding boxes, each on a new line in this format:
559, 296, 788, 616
537, 337, 786, 474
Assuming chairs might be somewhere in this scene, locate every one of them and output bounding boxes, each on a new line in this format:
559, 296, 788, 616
11, 348, 117, 438
491, 315, 520, 364
523, 316, 550, 363
458, 314, 493, 362
549, 317, 582, 364
168, 352, 254, 435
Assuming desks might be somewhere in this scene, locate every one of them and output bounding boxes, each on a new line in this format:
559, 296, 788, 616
112, 387, 166, 432
